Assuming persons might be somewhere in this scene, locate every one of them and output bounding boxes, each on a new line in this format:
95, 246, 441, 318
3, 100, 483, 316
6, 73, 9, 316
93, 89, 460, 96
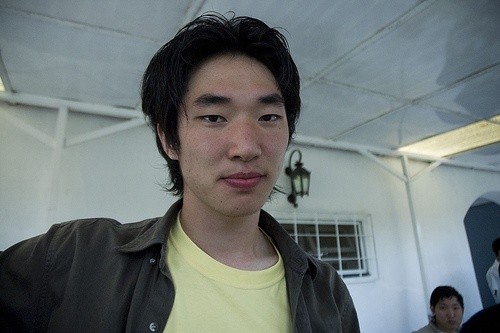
412, 286, 464, 333
486, 238, 500, 303
0, 9, 361, 333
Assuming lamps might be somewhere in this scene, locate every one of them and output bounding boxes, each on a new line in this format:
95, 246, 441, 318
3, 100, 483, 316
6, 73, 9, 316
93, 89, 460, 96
285, 150, 310, 208
